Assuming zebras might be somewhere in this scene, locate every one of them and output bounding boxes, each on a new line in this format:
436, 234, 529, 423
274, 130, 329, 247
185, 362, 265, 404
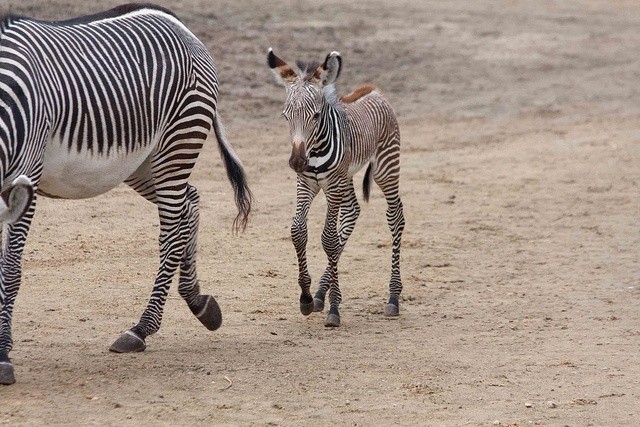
0, 2, 253, 385
268, 48, 405, 328
